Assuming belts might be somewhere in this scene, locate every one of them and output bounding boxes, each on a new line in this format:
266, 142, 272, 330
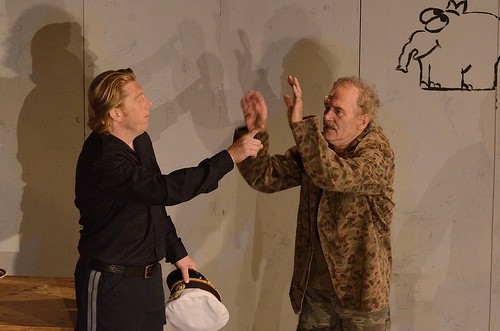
80, 257, 160, 279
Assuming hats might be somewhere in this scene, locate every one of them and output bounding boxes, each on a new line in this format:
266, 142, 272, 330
165, 267, 229, 331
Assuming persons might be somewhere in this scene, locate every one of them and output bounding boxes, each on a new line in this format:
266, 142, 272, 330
74, 67, 265, 331
233, 74, 396, 331
